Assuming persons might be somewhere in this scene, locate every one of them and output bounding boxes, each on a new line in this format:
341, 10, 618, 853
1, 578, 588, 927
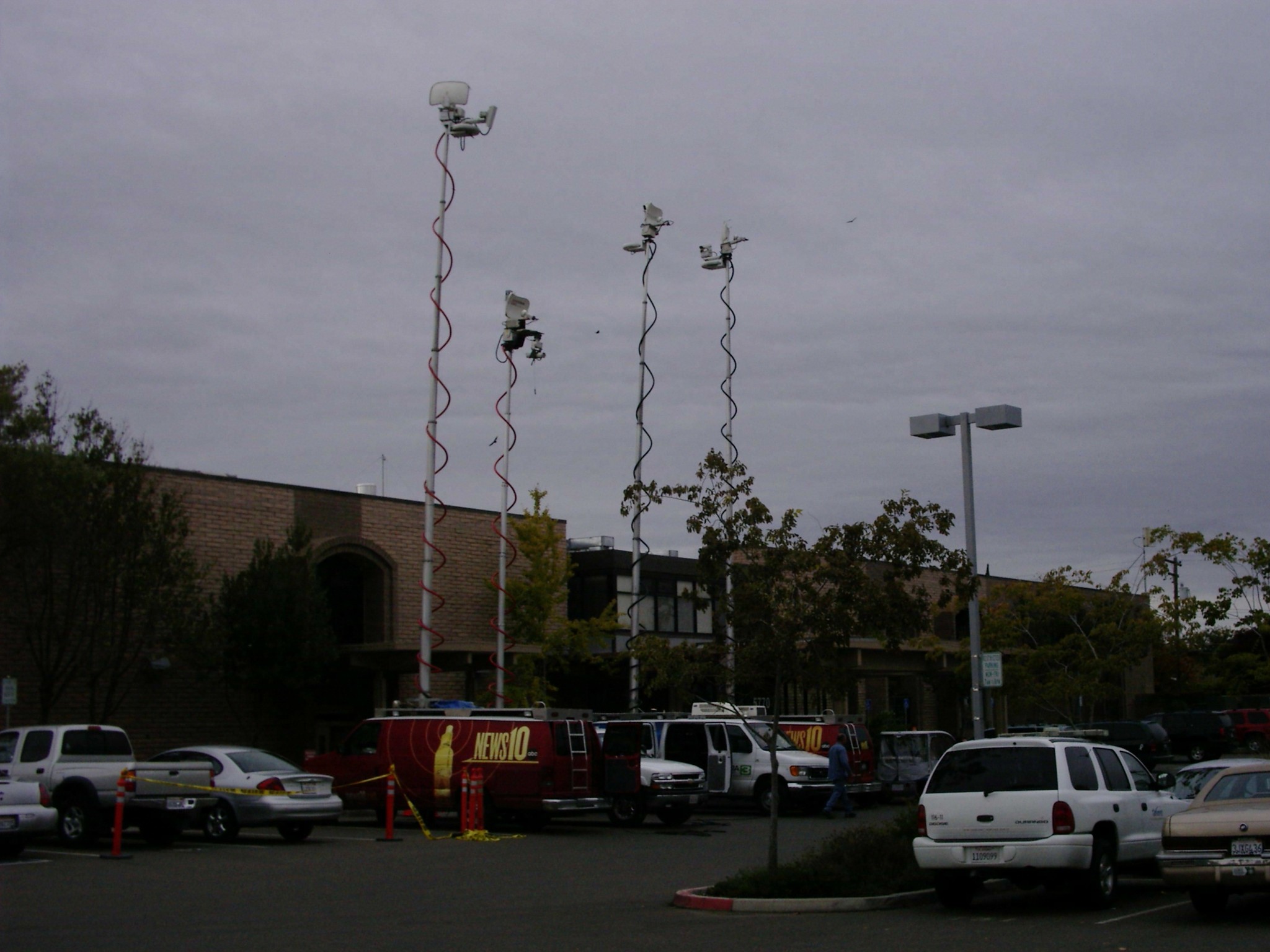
823, 734, 849, 816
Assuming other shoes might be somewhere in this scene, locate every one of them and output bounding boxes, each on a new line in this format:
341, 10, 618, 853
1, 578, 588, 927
845, 813, 856, 818
823, 809, 833, 819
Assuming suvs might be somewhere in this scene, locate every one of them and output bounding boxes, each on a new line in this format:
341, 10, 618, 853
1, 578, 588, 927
581, 699, 956, 833
910, 728, 1180, 914
295, 696, 645, 835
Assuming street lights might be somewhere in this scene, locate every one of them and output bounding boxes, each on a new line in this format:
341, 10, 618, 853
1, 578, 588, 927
909, 403, 1022, 740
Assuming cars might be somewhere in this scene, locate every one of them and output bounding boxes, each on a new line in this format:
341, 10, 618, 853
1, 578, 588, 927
0, 778, 60, 858
1065, 707, 1270, 814
1152, 762, 1270, 914
137, 743, 345, 845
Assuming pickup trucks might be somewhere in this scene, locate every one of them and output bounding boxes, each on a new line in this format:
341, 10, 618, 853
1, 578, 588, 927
0, 722, 219, 851
